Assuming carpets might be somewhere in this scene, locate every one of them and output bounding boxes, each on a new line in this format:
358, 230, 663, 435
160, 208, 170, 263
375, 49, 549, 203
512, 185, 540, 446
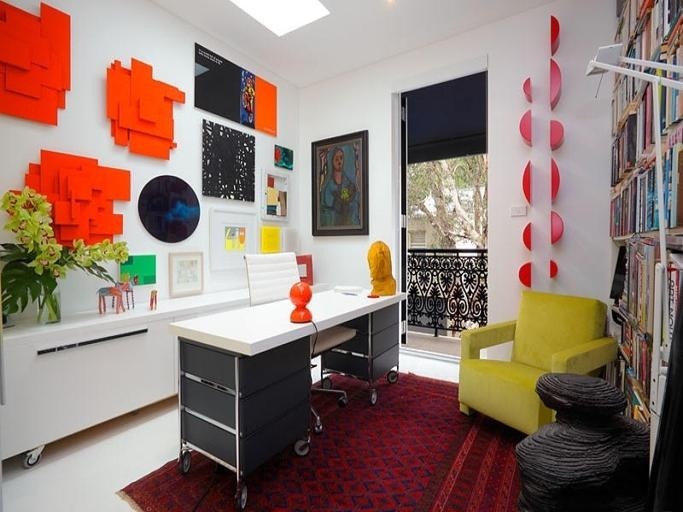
115, 368, 525, 511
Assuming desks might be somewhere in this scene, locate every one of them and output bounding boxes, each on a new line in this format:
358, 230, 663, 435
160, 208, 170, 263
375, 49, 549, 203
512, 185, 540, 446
171, 284, 410, 510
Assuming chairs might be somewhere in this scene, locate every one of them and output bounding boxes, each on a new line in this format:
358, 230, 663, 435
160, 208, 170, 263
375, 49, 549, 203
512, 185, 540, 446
454, 286, 618, 440
243, 249, 357, 433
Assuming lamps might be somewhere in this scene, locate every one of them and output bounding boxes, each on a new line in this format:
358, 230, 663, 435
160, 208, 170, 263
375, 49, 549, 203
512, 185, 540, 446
584, 42, 682, 92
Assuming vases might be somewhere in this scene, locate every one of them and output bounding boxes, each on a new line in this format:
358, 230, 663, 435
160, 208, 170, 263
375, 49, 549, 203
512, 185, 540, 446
34, 280, 62, 325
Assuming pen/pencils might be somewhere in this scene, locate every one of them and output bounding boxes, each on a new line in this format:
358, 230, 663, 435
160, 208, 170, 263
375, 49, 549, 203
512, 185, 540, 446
342, 292, 357, 295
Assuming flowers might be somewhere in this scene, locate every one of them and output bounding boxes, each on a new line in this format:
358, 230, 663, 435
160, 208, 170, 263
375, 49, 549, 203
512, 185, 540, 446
0, 185, 130, 325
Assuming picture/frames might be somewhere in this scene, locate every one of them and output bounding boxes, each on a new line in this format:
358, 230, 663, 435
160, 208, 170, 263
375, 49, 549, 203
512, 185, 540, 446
260, 168, 290, 226
167, 249, 204, 299
207, 207, 261, 274
309, 128, 370, 238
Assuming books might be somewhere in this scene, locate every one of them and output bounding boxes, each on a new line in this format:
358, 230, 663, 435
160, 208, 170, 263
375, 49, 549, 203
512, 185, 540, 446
604, 0, 683, 431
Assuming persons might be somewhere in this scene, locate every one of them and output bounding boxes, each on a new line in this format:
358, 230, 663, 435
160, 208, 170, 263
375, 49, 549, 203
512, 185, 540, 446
323, 148, 355, 226
368, 241, 396, 297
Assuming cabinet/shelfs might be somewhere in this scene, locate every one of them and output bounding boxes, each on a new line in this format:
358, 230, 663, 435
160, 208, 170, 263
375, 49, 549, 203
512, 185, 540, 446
607, 0, 682, 512
0, 289, 251, 464
